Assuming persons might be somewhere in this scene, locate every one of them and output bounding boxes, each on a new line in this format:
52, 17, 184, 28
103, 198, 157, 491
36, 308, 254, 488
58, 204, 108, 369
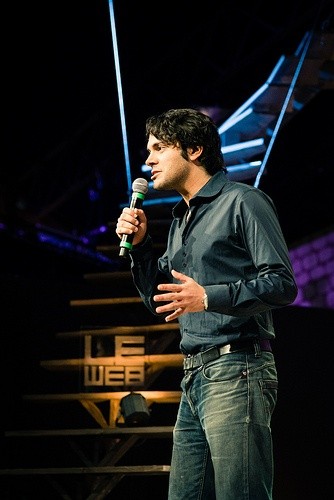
116, 110, 298, 500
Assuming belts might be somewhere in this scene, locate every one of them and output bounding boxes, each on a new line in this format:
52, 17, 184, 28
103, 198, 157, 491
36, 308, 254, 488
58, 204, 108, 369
181, 337, 270, 370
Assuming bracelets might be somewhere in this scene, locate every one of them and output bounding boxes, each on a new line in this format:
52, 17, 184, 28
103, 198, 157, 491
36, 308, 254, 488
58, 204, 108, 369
201, 294, 208, 310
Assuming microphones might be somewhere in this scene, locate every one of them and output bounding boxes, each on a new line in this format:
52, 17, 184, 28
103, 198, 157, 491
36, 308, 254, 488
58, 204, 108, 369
119, 178, 148, 258
